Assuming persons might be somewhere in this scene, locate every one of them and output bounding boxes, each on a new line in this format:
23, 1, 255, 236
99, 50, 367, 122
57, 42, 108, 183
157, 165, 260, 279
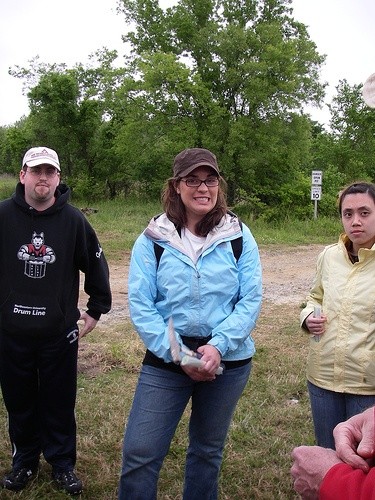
0, 146, 113, 496
289, 404, 375, 500
115, 147, 263, 500
299, 181, 375, 450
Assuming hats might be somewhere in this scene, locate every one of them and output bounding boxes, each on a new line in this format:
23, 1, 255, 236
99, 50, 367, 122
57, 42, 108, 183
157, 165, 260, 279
23, 147, 60, 171
174, 149, 221, 178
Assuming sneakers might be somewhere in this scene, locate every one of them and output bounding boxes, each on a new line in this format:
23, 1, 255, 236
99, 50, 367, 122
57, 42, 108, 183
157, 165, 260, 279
4, 466, 39, 489
52, 470, 82, 494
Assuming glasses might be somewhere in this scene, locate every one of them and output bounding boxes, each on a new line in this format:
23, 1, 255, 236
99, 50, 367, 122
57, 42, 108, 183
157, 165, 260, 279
178, 177, 222, 187
25, 168, 58, 176
